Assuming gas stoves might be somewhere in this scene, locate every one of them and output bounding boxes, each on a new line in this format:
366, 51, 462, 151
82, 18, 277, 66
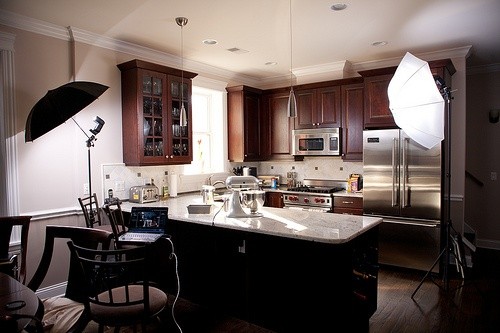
283, 178, 348, 208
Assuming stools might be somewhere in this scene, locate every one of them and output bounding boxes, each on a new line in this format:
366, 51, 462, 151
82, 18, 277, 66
0, 193, 172, 333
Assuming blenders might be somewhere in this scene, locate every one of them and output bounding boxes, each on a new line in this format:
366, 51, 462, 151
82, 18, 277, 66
225, 176, 265, 218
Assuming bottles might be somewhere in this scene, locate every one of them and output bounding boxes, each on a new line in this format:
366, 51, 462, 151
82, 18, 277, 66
143, 101, 162, 115
287, 166, 296, 189
271, 177, 275, 187
162, 170, 169, 200
144, 142, 188, 156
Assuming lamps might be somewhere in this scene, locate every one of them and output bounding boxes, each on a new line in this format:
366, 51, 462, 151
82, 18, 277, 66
175, 17, 188, 126
287, 0, 297, 118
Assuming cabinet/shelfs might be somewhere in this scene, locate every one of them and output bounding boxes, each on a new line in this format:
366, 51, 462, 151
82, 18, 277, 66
333, 196, 363, 216
224, 59, 457, 163
117, 59, 198, 166
263, 192, 285, 208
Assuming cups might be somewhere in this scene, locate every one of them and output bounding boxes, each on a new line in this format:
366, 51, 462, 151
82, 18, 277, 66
243, 168, 249, 176
202, 184, 215, 207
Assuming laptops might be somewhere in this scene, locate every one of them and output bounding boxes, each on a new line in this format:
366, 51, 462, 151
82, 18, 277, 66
118, 207, 168, 243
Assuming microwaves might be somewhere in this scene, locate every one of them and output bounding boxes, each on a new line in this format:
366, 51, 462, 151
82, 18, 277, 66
291, 127, 340, 155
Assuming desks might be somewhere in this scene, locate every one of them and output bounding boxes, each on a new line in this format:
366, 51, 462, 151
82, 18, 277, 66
0, 271, 44, 333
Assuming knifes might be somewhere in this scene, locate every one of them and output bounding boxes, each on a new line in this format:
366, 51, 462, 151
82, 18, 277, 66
233, 166, 246, 176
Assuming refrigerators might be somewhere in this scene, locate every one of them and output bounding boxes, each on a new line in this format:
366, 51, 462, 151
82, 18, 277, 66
362, 128, 442, 274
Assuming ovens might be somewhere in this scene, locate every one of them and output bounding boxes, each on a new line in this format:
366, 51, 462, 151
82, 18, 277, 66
284, 203, 330, 213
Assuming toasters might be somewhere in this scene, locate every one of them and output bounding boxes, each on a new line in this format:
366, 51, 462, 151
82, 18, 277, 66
130, 186, 160, 204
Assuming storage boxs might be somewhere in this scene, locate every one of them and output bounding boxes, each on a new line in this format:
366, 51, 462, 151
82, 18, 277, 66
187, 204, 212, 214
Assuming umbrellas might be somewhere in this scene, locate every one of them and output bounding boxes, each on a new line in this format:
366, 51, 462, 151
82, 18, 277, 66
386, 52, 444, 149
25, 81, 111, 142
